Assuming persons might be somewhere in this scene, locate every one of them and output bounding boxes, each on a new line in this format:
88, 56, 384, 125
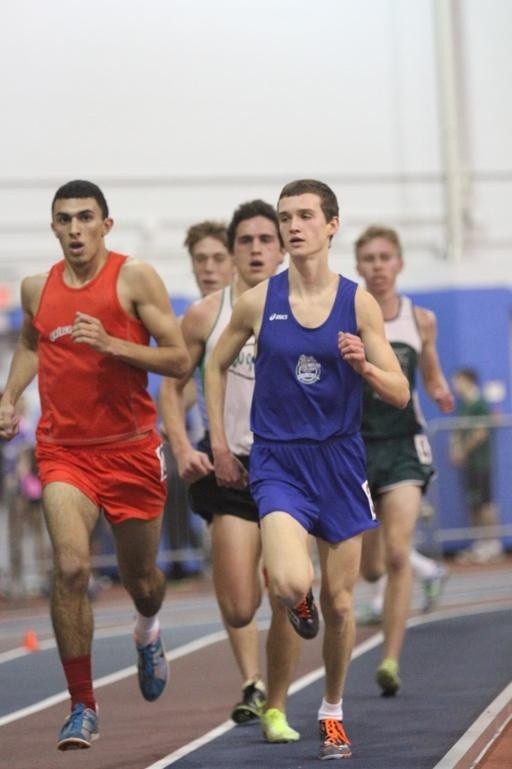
447, 370, 503, 564
156, 217, 267, 725
353, 223, 454, 694
160, 199, 302, 744
0, 179, 193, 751
203, 178, 411, 761
357, 546, 447, 626
1, 323, 208, 600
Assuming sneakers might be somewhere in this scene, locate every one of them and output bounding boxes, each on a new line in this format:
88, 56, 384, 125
231, 683, 268, 724
358, 586, 385, 625
316, 716, 352, 762
56, 701, 101, 752
418, 560, 451, 614
132, 623, 169, 702
258, 708, 302, 745
373, 659, 404, 697
285, 588, 320, 640
